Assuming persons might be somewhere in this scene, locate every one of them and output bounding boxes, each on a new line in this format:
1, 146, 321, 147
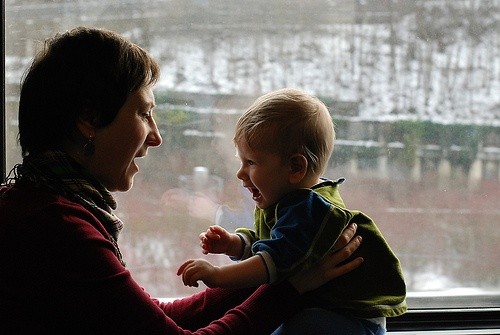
0, 27, 363, 335
175, 89, 386, 335
